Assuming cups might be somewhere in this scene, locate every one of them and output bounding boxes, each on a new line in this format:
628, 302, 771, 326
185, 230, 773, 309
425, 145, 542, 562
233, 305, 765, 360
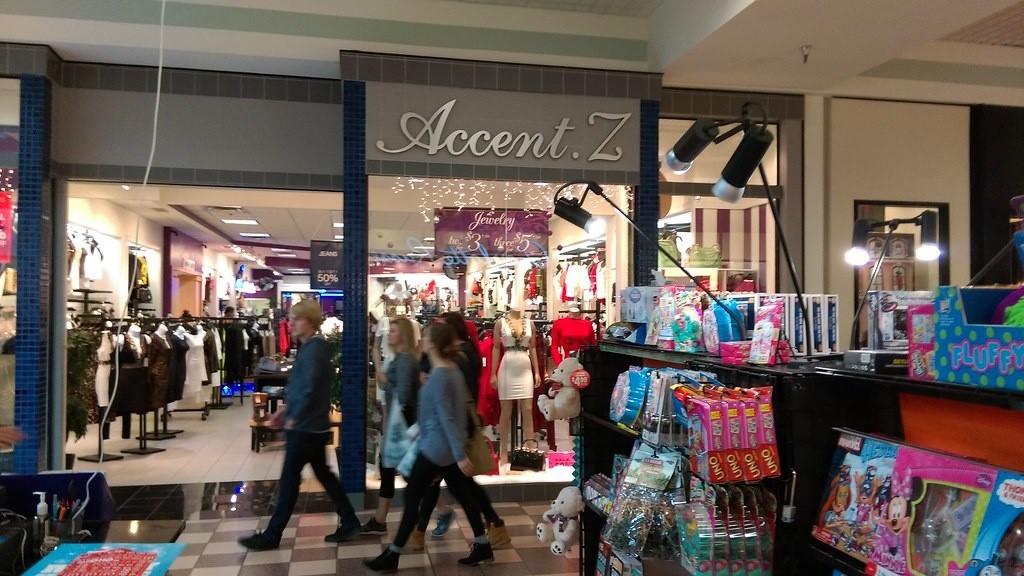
52, 516, 82, 543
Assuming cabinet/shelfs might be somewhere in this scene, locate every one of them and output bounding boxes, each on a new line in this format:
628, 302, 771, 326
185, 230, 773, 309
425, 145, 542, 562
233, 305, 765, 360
580, 342, 1023, 575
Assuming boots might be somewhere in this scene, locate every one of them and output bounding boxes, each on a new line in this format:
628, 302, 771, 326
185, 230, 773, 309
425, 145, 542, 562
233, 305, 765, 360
457, 542, 495, 566
361, 545, 400, 573
486, 519, 511, 549
400, 528, 425, 555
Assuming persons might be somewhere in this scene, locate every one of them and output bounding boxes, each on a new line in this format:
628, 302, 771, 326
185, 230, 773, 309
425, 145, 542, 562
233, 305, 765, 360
492, 305, 541, 468
66, 231, 259, 417
363, 321, 494, 572
222, 307, 235, 323
373, 285, 423, 440
318, 310, 343, 339
278, 310, 292, 357
402, 312, 512, 552
237, 301, 361, 550
595, 243, 607, 302
357, 318, 455, 535
551, 308, 597, 367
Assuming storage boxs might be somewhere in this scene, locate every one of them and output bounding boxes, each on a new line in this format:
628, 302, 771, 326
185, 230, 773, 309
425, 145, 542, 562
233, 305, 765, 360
617, 287, 1023, 479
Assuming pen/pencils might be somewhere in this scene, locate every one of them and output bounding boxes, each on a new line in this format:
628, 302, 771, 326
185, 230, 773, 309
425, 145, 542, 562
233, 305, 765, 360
52, 494, 81, 536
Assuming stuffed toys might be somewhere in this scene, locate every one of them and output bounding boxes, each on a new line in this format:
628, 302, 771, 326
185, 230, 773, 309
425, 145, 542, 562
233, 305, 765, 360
536, 487, 585, 556
537, 358, 584, 421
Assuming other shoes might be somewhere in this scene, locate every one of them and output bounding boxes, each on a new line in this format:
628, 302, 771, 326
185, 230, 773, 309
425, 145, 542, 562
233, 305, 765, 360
324, 516, 361, 542
360, 515, 388, 535
431, 508, 456, 537
237, 533, 282, 550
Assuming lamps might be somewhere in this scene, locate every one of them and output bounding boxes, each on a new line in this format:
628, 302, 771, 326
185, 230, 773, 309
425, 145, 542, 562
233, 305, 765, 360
665, 98, 812, 359
845, 211, 942, 350
554, 179, 745, 339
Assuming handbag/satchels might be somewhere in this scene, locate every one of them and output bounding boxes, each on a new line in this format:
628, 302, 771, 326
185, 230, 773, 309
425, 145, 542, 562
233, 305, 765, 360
380, 389, 413, 468
662, 229, 683, 265
658, 239, 677, 267
510, 439, 543, 472
467, 432, 495, 474
0, 507, 33, 575
397, 431, 421, 477
131, 287, 152, 302
479, 436, 500, 475
688, 242, 723, 268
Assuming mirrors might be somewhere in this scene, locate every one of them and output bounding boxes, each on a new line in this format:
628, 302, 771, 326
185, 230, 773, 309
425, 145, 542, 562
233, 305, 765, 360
853, 199, 949, 350
